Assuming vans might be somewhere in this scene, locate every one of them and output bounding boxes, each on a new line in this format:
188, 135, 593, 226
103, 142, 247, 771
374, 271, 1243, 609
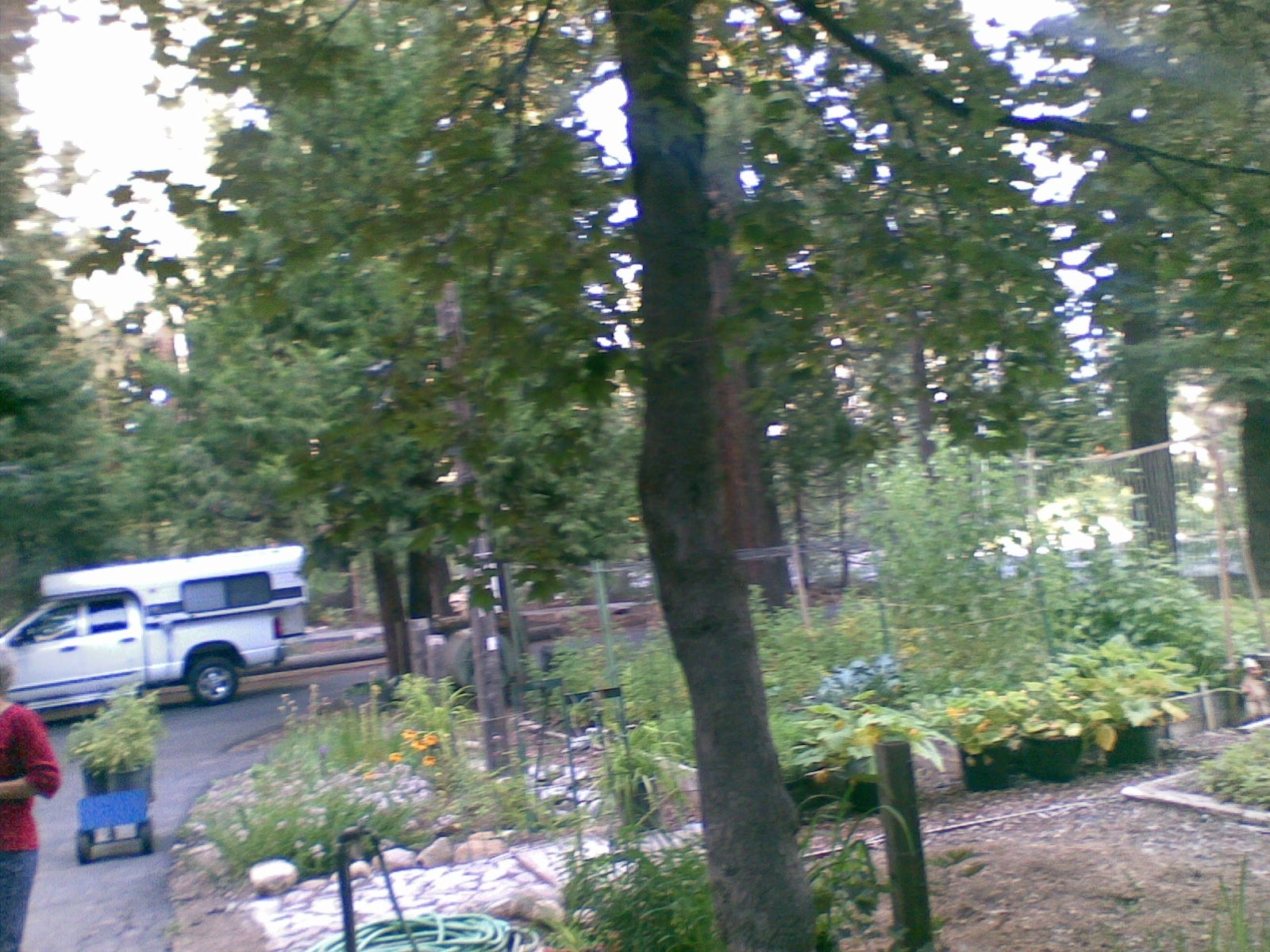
1, 544, 309, 713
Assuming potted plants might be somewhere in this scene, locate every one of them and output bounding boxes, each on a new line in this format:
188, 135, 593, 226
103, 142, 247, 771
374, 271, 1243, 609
815, 698, 943, 811
68, 693, 160, 805
914, 694, 1031, 789
1064, 634, 1190, 780
767, 715, 844, 809
1005, 676, 1093, 776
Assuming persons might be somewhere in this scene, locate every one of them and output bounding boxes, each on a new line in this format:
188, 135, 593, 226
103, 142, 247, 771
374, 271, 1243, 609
1238, 657, 1269, 725
0, 642, 61, 952
